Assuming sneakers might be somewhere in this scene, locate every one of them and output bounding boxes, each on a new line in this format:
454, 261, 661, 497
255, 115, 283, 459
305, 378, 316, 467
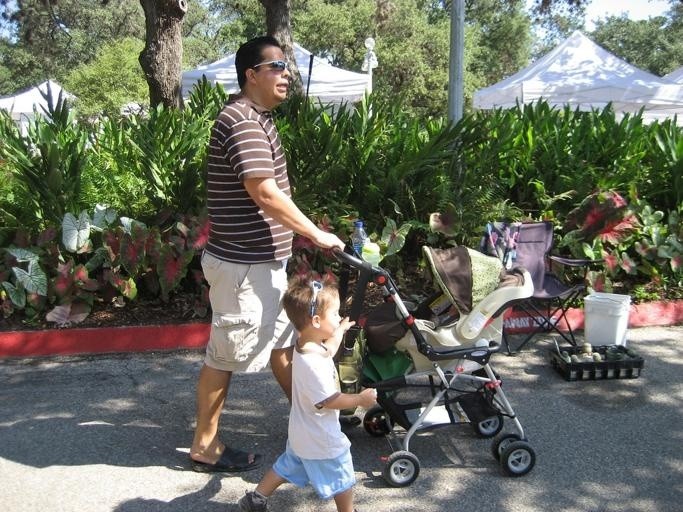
237, 491, 271, 512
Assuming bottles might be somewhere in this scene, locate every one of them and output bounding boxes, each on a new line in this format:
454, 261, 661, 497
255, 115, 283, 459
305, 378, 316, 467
352, 220, 380, 268
339, 338, 360, 383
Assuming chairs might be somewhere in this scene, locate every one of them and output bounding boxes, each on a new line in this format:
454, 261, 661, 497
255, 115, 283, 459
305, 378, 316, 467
482, 221, 606, 356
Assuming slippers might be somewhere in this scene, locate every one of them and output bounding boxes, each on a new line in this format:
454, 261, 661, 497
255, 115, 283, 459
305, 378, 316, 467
192, 444, 264, 473
339, 416, 362, 432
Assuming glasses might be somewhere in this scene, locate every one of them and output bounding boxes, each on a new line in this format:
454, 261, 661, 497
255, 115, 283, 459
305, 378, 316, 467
309, 281, 323, 318
251, 59, 289, 70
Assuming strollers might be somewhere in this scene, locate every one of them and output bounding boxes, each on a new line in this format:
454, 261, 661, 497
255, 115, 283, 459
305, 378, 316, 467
326, 246, 536, 487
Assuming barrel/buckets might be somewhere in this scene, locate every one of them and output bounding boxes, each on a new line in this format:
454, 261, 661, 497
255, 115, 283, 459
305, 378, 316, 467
582, 292, 632, 346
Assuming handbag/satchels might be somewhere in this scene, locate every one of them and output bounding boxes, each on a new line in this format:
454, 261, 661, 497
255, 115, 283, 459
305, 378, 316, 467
331, 346, 362, 413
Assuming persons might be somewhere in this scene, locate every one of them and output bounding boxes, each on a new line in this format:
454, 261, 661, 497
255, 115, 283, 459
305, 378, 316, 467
237, 268, 378, 512
188, 37, 363, 472
